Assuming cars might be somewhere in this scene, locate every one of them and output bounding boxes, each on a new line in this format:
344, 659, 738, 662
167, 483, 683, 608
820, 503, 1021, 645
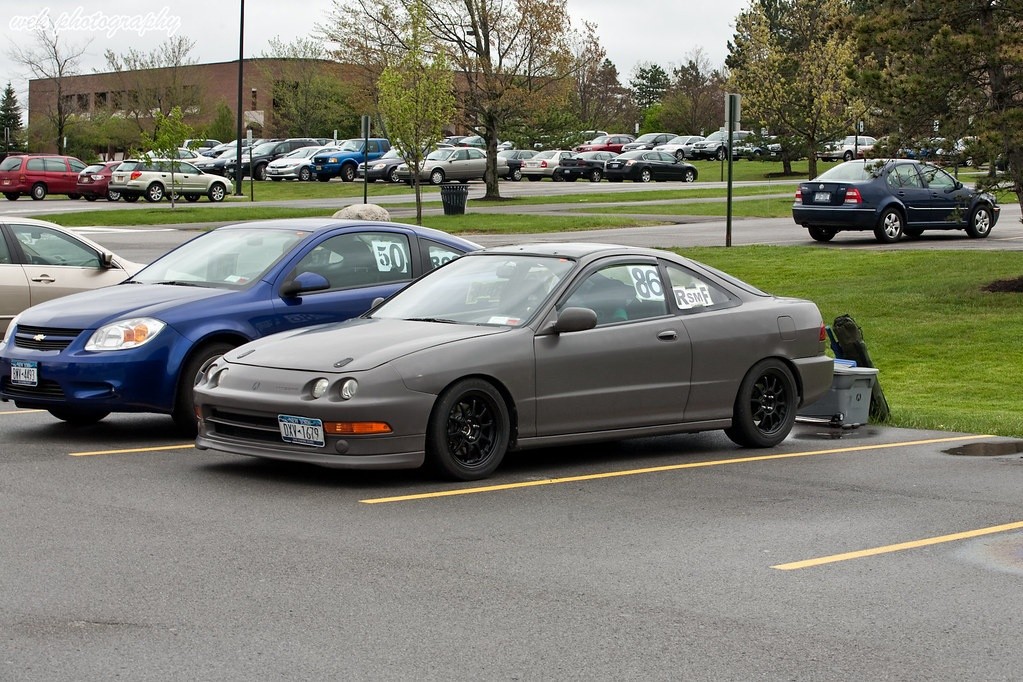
394, 146, 510, 185
557, 150, 620, 183
178, 136, 333, 180
1, 217, 507, 437
620, 133, 679, 154
575, 136, 636, 155
76, 160, 124, 202
0, 216, 205, 339
267, 146, 341, 182
736, 134, 1011, 170
499, 150, 548, 182
519, 149, 579, 182
603, 150, 699, 184
791, 157, 1003, 244
355, 146, 431, 184
437, 130, 608, 152
140, 148, 215, 167
194, 242, 836, 483
651, 135, 705, 161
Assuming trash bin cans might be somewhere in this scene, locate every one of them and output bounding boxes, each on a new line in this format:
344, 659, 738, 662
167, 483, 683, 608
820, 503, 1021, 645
438, 183, 470, 215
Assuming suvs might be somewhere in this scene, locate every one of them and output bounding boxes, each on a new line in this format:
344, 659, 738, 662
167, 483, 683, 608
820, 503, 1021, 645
691, 130, 755, 161
310, 138, 391, 183
0, 154, 89, 201
109, 157, 235, 204
